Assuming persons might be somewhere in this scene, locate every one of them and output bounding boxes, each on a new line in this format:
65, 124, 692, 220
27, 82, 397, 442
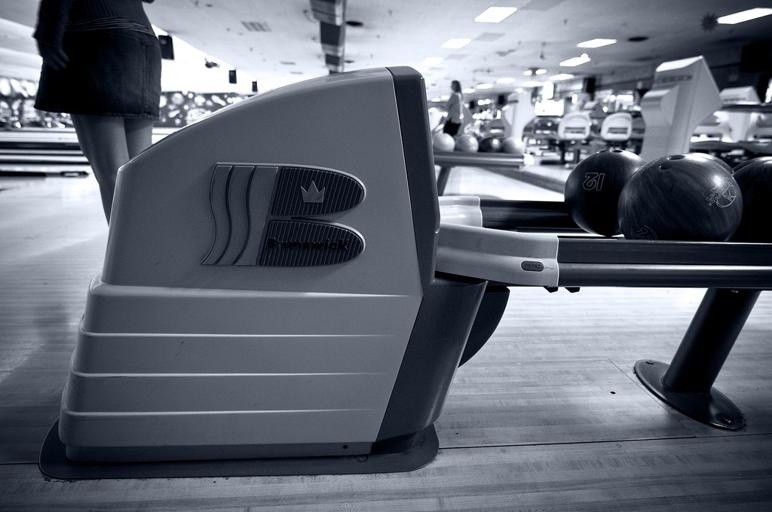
439, 80, 463, 136
32, 1, 163, 226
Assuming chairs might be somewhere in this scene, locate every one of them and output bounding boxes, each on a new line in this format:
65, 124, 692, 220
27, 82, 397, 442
524, 112, 645, 170
